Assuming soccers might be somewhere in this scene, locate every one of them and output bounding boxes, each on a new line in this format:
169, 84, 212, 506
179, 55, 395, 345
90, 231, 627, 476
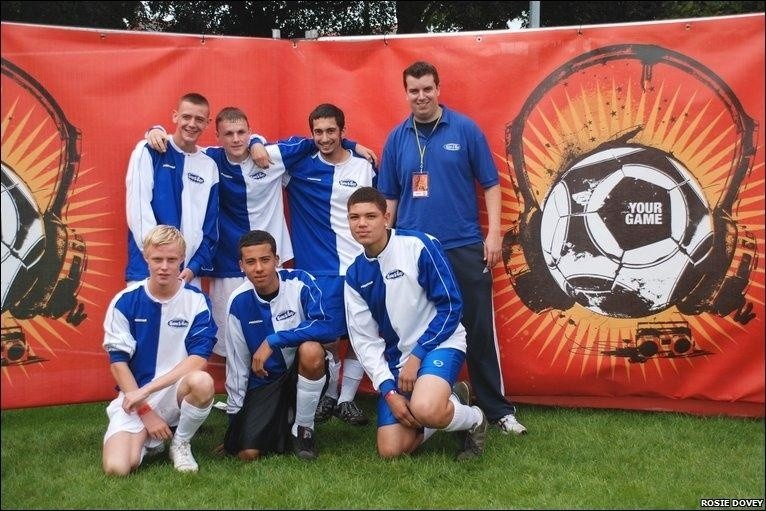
541, 147, 714, 318
0, 164, 47, 313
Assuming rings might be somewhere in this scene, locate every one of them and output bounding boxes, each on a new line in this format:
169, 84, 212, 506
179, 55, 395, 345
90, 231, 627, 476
157, 435, 162, 441
493, 255, 496, 257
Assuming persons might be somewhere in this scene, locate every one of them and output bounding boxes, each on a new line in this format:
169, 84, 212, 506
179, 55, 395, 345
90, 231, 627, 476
100, 224, 217, 478
343, 186, 487, 465
210, 229, 336, 462
142, 106, 377, 361
124, 91, 221, 292
377, 61, 528, 437
247, 102, 378, 427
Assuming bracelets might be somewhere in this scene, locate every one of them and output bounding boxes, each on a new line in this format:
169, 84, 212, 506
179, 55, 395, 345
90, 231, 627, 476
135, 404, 150, 416
384, 389, 396, 402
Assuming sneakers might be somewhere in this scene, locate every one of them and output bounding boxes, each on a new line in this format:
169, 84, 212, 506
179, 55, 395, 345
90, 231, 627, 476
451, 379, 472, 405
332, 400, 369, 424
314, 394, 337, 420
287, 423, 317, 459
496, 413, 527, 435
455, 405, 487, 461
168, 438, 198, 472
